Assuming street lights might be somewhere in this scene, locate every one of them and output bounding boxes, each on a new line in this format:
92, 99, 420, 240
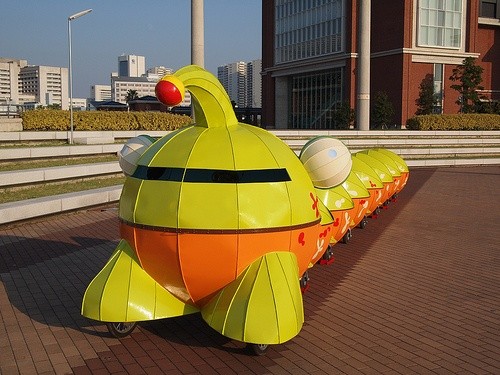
66, 9, 93, 145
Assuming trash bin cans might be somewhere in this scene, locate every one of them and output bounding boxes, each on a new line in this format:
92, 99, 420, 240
430, 104, 442, 114
